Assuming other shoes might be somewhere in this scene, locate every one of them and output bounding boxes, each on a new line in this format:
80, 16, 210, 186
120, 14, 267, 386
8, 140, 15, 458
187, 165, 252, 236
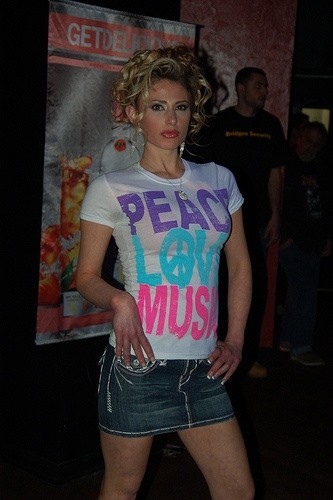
250, 362, 274, 379
279, 341, 294, 352
291, 351, 325, 365
